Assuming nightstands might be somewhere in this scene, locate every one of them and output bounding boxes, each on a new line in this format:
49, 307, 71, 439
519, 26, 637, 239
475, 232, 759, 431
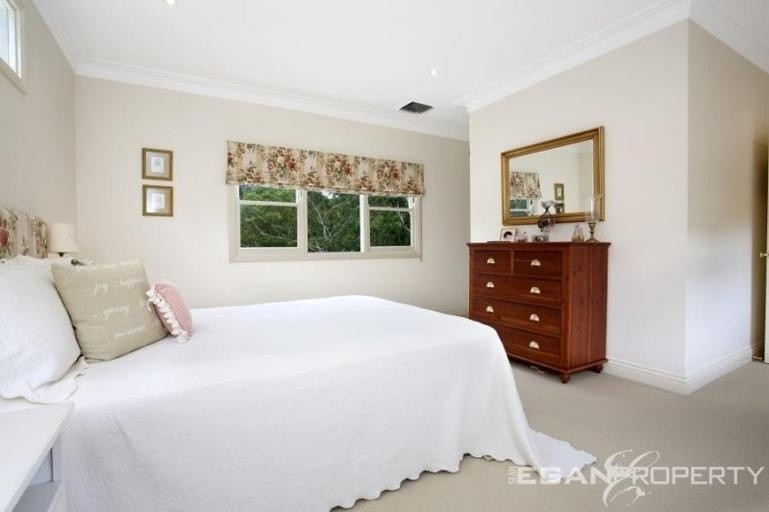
0, 395, 77, 511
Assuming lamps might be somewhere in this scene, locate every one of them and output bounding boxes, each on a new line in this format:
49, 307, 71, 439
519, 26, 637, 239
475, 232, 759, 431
45, 222, 76, 257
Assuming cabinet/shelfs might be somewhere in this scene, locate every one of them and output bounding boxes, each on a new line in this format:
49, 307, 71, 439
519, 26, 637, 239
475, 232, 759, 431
465, 241, 612, 385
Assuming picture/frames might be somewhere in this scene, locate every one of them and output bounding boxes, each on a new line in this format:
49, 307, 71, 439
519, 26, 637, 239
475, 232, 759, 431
141, 146, 174, 218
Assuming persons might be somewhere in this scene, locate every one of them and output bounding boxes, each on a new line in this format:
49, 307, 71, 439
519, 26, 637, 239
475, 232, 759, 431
503, 231, 512, 240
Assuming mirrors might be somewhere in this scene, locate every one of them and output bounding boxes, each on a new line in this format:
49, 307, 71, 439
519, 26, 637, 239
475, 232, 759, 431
498, 126, 606, 226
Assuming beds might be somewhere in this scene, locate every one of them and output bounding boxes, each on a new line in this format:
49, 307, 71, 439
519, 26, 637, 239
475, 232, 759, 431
1, 203, 512, 512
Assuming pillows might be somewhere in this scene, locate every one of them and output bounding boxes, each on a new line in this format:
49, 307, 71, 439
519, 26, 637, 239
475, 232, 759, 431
0, 251, 193, 407
